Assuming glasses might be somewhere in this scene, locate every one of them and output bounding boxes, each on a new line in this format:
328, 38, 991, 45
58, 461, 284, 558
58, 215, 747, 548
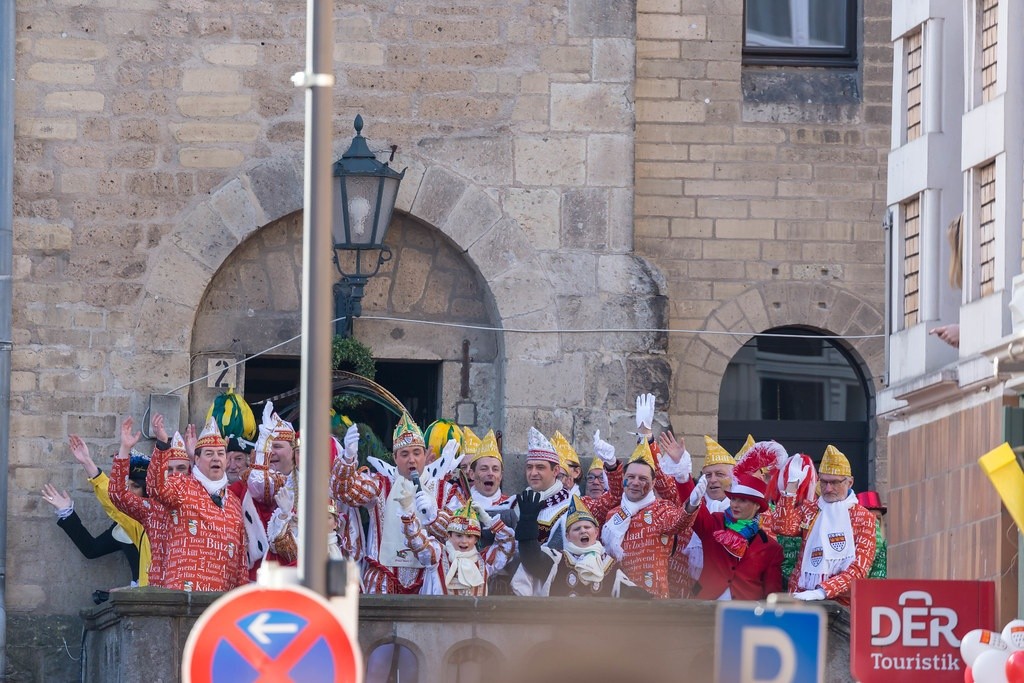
568, 464, 577, 468
212, 495, 221, 506
586, 473, 605, 483
820, 479, 848, 485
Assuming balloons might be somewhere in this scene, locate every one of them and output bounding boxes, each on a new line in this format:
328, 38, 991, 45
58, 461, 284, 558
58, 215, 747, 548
960, 618, 1024, 683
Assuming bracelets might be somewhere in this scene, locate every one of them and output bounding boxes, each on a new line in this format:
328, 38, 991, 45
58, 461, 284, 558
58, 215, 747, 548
780, 490, 796, 495
627, 430, 652, 437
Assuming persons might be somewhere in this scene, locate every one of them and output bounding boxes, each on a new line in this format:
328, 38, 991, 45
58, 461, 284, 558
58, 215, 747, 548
37, 392, 886, 601
928, 213, 962, 348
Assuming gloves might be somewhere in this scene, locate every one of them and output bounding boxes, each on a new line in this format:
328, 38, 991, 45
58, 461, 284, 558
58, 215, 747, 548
391, 482, 413, 513
690, 474, 708, 506
343, 423, 360, 459
636, 393, 655, 429
594, 429, 616, 465
415, 492, 432, 518
793, 587, 826, 601
471, 502, 492, 526
788, 453, 810, 488
515, 491, 545, 541
261, 401, 278, 433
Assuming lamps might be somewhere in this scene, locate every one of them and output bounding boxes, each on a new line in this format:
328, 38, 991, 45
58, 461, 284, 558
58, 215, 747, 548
332, 114, 409, 337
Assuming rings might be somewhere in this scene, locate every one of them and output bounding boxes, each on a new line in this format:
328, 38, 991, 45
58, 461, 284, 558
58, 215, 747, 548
50, 498, 53, 500
154, 425, 157, 426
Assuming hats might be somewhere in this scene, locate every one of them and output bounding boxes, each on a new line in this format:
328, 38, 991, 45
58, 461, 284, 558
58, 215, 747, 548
856, 492, 887, 515
724, 441, 788, 513
702, 435, 736, 467
818, 445, 851, 476
550, 430, 581, 477
129, 385, 559, 542
627, 436, 656, 472
588, 454, 604, 473
734, 434, 755, 460
567, 494, 599, 529
